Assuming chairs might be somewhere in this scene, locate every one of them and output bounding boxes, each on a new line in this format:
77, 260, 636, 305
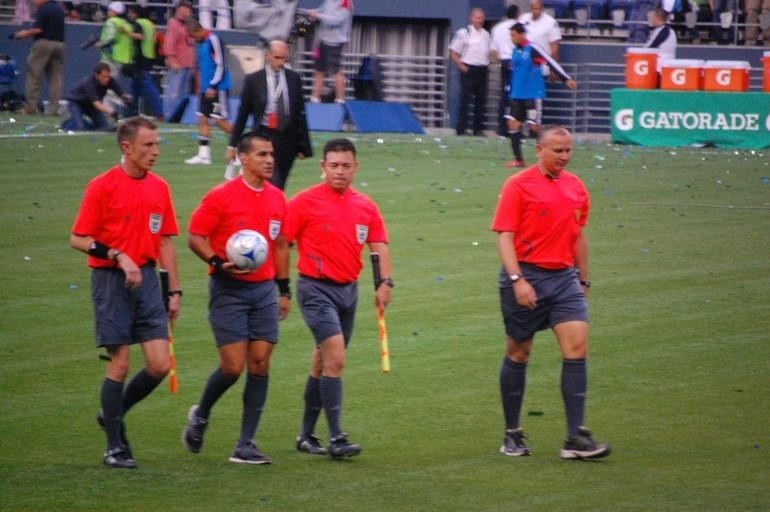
542, 0, 735, 45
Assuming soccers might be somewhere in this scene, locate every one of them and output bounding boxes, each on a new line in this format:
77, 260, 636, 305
225, 229, 268, 270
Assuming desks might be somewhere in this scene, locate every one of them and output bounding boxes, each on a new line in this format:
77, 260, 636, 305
610, 88, 770, 149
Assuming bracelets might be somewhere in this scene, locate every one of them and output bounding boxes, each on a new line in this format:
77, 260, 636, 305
580, 279, 592, 289
209, 254, 224, 273
87, 240, 111, 261
381, 277, 393, 288
166, 289, 182, 298
112, 248, 120, 262
277, 277, 291, 298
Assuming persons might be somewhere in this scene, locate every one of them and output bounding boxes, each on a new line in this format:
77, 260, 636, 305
226, 39, 314, 191
95, 1, 194, 124
68, 115, 185, 470
288, 138, 394, 460
448, 0, 562, 138
14, 0, 65, 116
491, 123, 611, 458
502, 22, 577, 168
628, 0, 770, 47
62, 63, 133, 132
185, 130, 292, 467
645, 8, 677, 71
183, 16, 235, 166
307, 0, 354, 104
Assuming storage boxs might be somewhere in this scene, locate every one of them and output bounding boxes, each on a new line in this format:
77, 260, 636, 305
660, 59, 705, 90
759, 51, 770, 92
703, 60, 751, 92
623, 47, 659, 90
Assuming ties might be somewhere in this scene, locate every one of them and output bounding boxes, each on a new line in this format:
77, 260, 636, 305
273, 73, 287, 134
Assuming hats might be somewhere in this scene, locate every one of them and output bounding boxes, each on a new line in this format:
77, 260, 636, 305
108, 1, 127, 14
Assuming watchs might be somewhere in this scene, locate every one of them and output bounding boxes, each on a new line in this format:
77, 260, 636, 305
510, 272, 522, 284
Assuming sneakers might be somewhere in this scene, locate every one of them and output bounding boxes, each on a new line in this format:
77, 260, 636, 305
95, 407, 137, 469
182, 404, 208, 453
560, 425, 609, 460
499, 430, 529, 457
14, 106, 39, 116
503, 159, 523, 167
457, 129, 487, 136
229, 439, 272, 465
295, 433, 328, 454
184, 155, 213, 165
326, 431, 362, 459
